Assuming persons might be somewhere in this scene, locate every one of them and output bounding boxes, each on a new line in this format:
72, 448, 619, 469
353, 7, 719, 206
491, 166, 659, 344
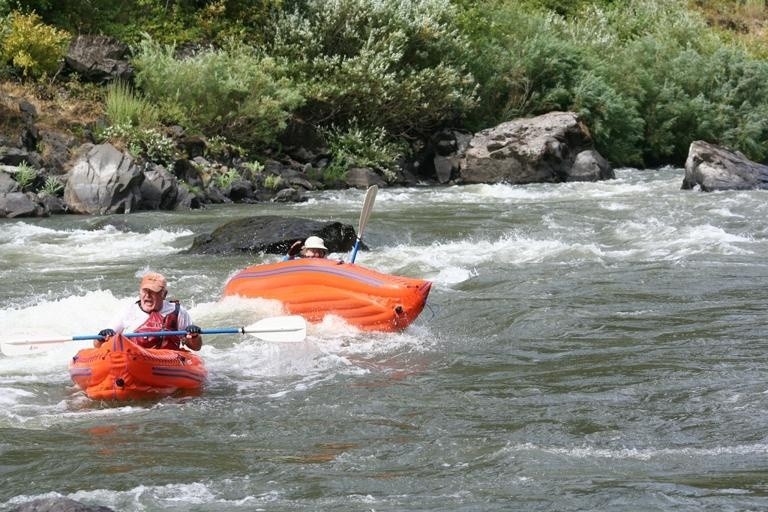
92, 270, 203, 351
281, 236, 344, 265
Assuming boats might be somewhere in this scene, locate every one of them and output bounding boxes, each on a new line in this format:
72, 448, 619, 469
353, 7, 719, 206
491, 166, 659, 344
214, 258, 433, 336
65, 338, 206, 405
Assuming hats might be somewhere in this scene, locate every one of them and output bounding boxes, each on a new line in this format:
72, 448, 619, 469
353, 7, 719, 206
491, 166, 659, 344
304, 236, 327, 250
142, 273, 167, 293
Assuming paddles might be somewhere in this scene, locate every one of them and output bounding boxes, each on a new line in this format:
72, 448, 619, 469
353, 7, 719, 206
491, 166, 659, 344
0, 315, 306, 356
350, 185, 376, 264
